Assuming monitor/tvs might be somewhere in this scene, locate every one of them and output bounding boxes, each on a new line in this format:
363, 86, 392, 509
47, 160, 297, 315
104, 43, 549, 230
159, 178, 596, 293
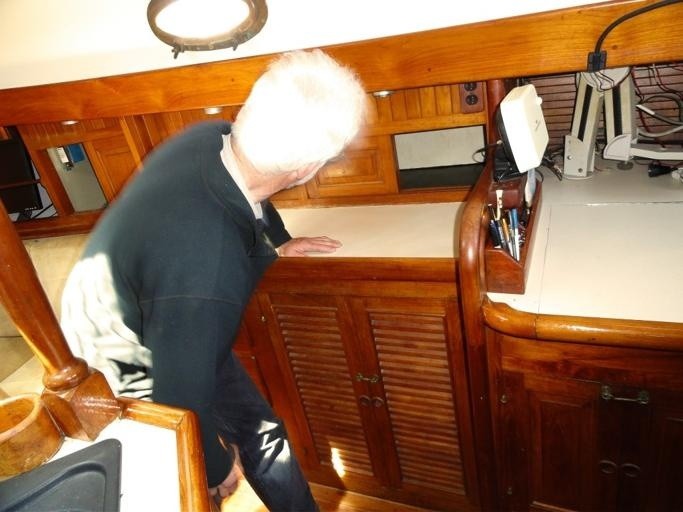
0, 138, 43, 221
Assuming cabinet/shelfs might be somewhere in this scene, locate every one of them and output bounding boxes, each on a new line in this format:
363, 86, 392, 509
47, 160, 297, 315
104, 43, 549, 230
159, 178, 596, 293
261, 292, 479, 503
521, 374, 683, 512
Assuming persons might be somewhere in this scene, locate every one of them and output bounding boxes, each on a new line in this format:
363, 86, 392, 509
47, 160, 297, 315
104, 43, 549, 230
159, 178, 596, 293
56, 45, 366, 511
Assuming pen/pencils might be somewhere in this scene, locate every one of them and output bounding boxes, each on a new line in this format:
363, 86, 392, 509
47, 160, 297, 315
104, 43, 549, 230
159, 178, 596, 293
489, 190, 520, 262
